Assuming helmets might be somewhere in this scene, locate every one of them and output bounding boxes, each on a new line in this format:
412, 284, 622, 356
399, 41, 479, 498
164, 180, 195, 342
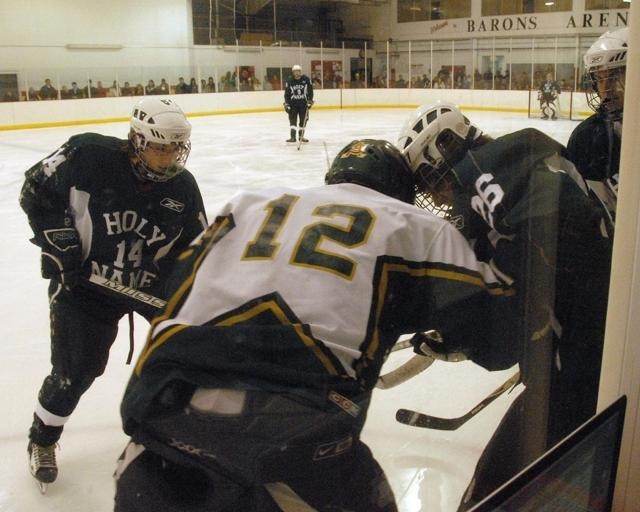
291, 64, 303, 80
319, 136, 417, 208
395, 97, 486, 225
127, 94, 195, 185
580, 26, 629, 124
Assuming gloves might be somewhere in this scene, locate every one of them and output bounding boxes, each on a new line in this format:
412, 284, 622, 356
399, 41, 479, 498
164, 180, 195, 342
28, 212, 85, 280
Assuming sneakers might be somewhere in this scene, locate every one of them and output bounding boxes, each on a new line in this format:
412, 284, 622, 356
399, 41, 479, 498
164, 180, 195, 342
24, 437, 60, 483
286, 136, 310, 142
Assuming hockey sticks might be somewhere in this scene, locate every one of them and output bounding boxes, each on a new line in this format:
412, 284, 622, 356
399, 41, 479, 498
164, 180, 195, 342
296, 108, 309, 150
79, 267, 442, 388
396, 371, 523, 431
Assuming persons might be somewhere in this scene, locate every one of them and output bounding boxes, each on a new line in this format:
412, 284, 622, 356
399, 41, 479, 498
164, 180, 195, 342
284, 65, 313, 142
239, 68, 281, 91
122, 77, 169, 97
110, 140, 522, 512
314, 68, 588, 92
60, 79, 122, 98
176, 71, 237, 94
537, 73, 561, 119
20, 99, 208, 483
396, 102, 613, 512
5, 78, 58, 99
567, 27, 627, 218
